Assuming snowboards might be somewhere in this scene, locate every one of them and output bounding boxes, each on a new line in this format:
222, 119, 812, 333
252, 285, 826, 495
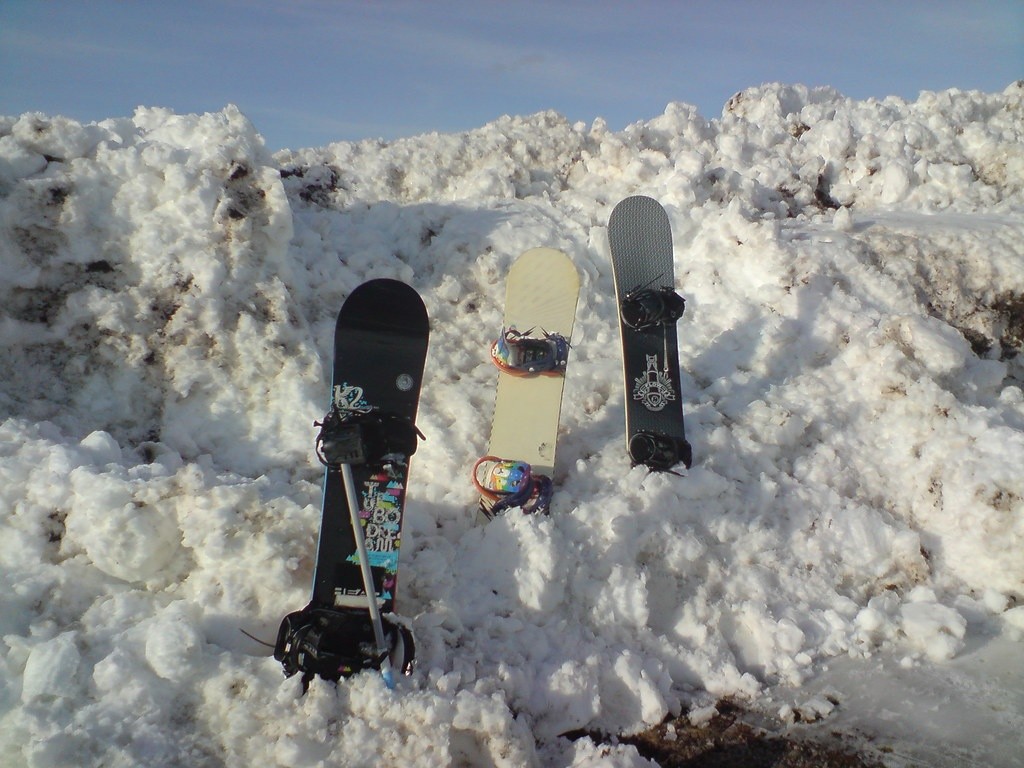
609, 194, 694, 475
274, 277, 432, 692
480, 246, 579, 521
